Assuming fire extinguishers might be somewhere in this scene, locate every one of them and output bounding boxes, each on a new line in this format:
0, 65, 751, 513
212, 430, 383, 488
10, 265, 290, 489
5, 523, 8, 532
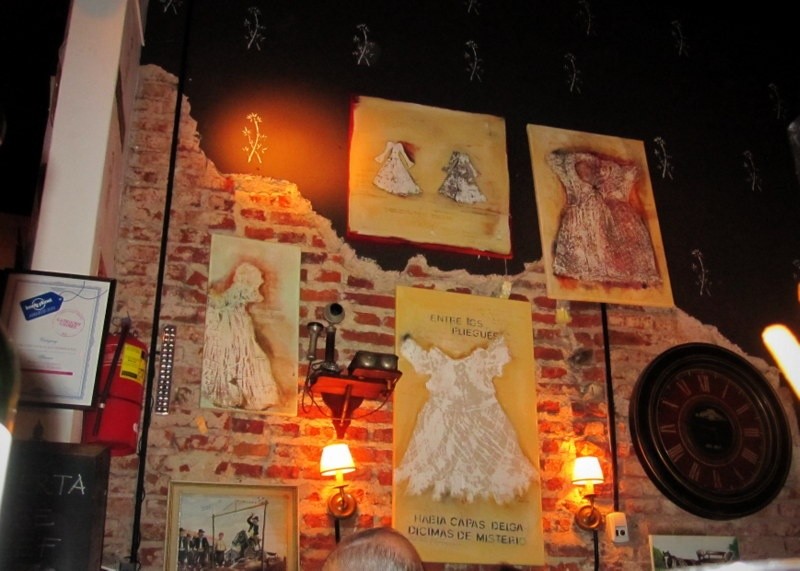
76, 310, 148, 456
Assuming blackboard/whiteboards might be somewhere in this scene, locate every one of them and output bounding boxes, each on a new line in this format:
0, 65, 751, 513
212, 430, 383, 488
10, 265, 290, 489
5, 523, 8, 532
0, 440, 112, 571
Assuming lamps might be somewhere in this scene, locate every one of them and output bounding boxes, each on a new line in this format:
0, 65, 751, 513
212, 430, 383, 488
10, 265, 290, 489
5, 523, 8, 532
570, 456, 605, 532
320, 442, 358, 518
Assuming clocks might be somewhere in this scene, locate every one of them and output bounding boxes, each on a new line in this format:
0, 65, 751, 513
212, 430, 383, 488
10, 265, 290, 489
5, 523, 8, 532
628, 341, 793, 525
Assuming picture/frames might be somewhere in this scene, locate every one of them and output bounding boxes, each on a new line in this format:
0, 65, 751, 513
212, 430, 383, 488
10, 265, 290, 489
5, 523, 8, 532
161, 479, 300, 571
